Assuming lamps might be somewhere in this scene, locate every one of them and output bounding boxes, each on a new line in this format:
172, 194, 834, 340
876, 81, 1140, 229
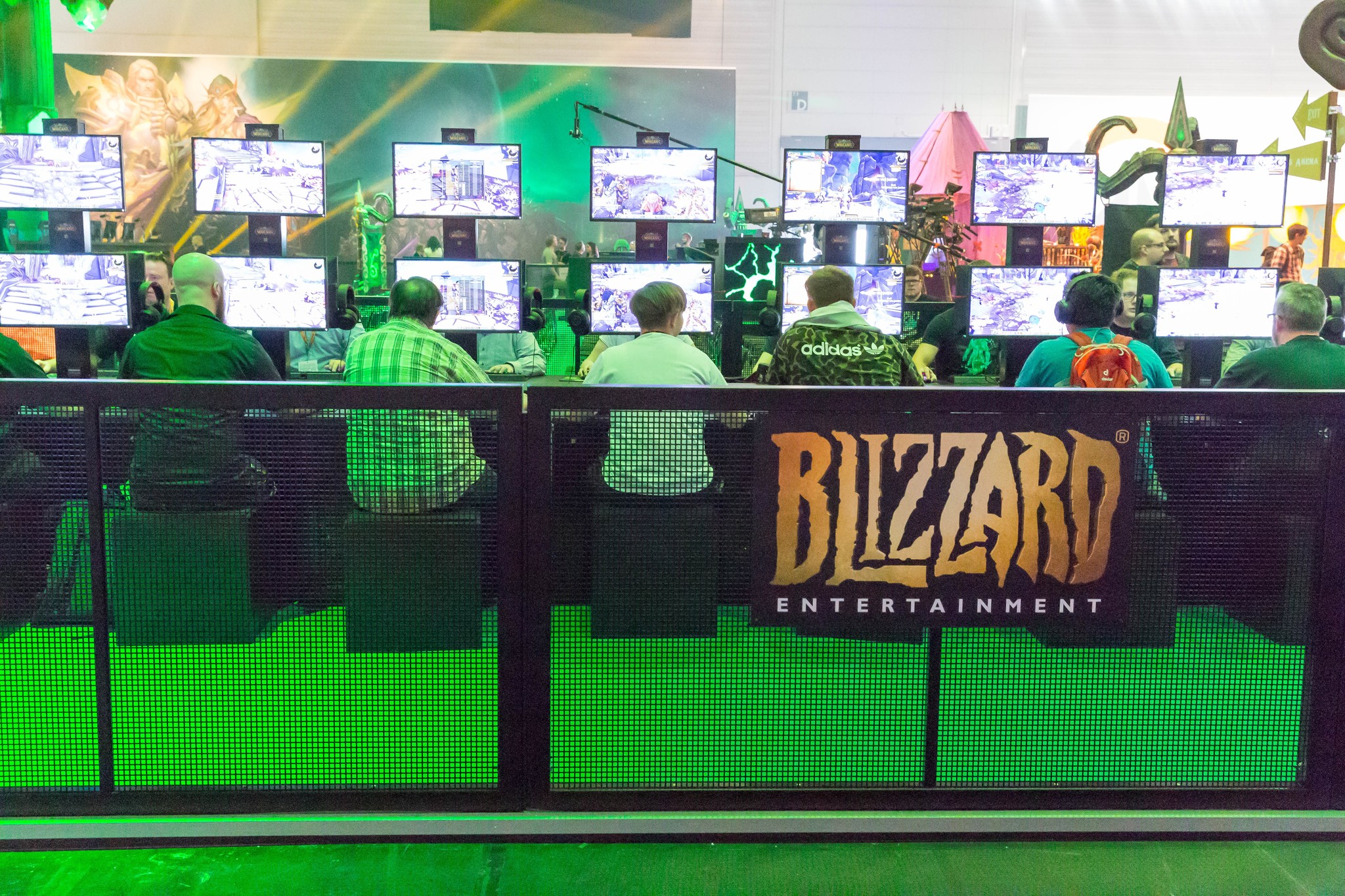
944, 182, 962, 199
7, 219, 19, 244
38, 221, 50, 244
90, 214, 140, 242
909, 183, 923, 202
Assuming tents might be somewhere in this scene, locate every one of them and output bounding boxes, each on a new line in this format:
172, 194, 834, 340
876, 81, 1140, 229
910, 111, 1058, 266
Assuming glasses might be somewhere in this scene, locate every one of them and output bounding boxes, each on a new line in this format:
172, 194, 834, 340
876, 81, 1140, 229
1124, 293, 1139, 300
1147, 243, 1165, 249
1267, 313, 1284, 321
906, 280, 924, 284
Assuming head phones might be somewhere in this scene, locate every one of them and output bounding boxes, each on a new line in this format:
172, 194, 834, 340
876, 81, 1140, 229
1054, 273, 1124, 325
523, 286, 546, 332
138, 281, 170, 329
1130, 294, 1156, 339
336, 283, 360, 330
568, 289, 591, 336
1324, 296, 1345, 335
759, 290, 780, 336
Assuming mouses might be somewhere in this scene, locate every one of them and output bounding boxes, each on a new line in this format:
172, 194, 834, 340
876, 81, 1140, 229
581, 370, 589, 380
922, 372, 932, 382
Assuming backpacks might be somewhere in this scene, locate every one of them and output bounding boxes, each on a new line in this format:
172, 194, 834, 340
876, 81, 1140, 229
1060, 331, 1150, 389
1261, 245, 1289, 273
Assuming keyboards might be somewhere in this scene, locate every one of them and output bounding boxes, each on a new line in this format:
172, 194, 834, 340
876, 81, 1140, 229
97, 368, 119, 377
290, 371, 343, 380
948, 374, 999, 383
485, 372, 527, 382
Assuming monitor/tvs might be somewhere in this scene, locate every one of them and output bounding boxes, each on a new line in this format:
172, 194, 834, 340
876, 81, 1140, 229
391, 142, 522, 220
970, 151, 1099, 227
0, 133, 126, 212
191, 137, 327, 217
1158, 153, 1290, 227
779, 263, 906, 338
1135, 265, 1279, 341
589, 146, 717, 224
953, 265, 1093, 340
782, 149, 910, 225
394, 256, 527, 334
1317, 267, 1345, 318
564, 251, 714, 336
206, 254, 339, 332
0, 251, 145, 331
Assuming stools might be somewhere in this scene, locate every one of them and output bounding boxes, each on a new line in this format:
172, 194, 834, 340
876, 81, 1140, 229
341, 506, 483, 651
1225, 515, 1316, 645
593, 504, 719, 638
1026, 511, 1176, 649
108, 511, 274, 644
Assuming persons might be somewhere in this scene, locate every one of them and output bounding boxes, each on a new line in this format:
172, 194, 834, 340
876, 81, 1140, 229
116, 213, 1345, 617
144, 252, 179, 314
0, 332, 65, 578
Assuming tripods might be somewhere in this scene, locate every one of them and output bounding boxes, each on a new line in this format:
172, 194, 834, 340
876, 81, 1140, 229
913, 217, 956, 302
